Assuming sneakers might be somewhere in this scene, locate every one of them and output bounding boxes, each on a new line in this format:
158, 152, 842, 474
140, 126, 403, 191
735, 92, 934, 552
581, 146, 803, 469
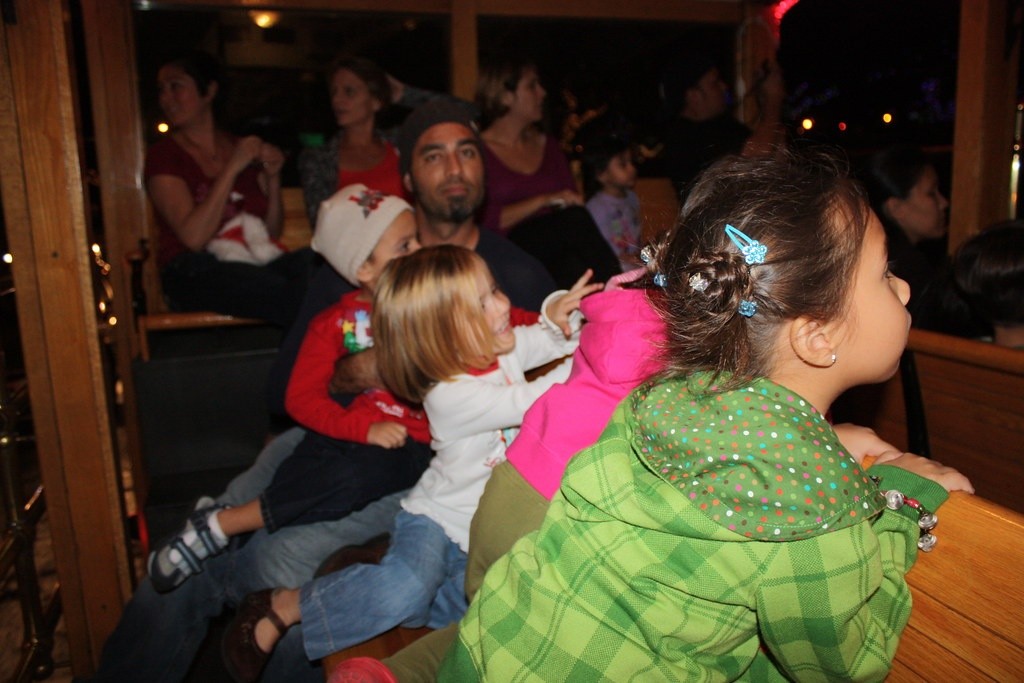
147, 498, 231, 594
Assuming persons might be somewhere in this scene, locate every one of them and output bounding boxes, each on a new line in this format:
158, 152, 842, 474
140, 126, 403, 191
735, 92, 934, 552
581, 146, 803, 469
431, 148, 974, 683
326, 229, 671, 683
222, 245, 605, 683
141, 44, 1024, 354
72, 103, 556, 683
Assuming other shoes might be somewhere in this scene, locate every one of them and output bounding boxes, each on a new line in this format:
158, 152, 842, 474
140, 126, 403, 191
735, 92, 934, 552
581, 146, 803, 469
327, 656, 398, 683
239, 585, 290, 669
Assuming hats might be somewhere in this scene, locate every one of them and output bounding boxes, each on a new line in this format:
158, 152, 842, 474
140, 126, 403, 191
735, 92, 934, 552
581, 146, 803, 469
311, 185, 413, 285
206, 215, 287, 267
394, 98, 482, 179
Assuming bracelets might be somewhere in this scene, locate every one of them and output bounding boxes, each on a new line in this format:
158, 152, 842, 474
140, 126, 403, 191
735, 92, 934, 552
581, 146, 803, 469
880, 489, 937, 553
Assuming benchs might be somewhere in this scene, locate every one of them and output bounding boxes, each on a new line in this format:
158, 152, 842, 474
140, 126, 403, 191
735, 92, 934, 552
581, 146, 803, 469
133, 178, 1024, 683
